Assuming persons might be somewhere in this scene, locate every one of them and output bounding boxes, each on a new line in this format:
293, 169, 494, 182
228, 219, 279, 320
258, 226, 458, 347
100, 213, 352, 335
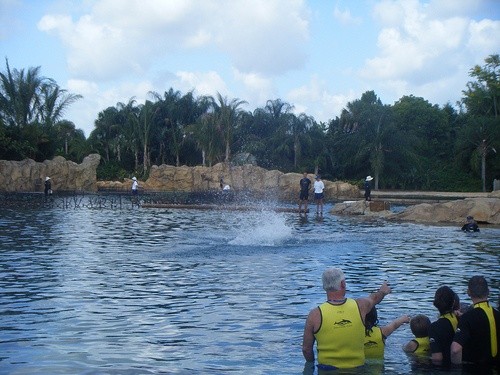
365, 176, 373, 201
298, 173, 312, 214
132, 177, 138, 196
314, 175, 324, 214
461, 216, 480, 232
403, 276, 500, 375
223, 185, 231, 190
302, 268, 391, 375
364, 306, 411, 375
44, 177, 53, 202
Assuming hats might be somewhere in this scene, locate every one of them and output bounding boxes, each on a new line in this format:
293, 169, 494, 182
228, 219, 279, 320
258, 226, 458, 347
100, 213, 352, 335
467, 216, 473, 220
45, 177, 51, 180
132, 177, 136, 180
365, 176, 373, 181
